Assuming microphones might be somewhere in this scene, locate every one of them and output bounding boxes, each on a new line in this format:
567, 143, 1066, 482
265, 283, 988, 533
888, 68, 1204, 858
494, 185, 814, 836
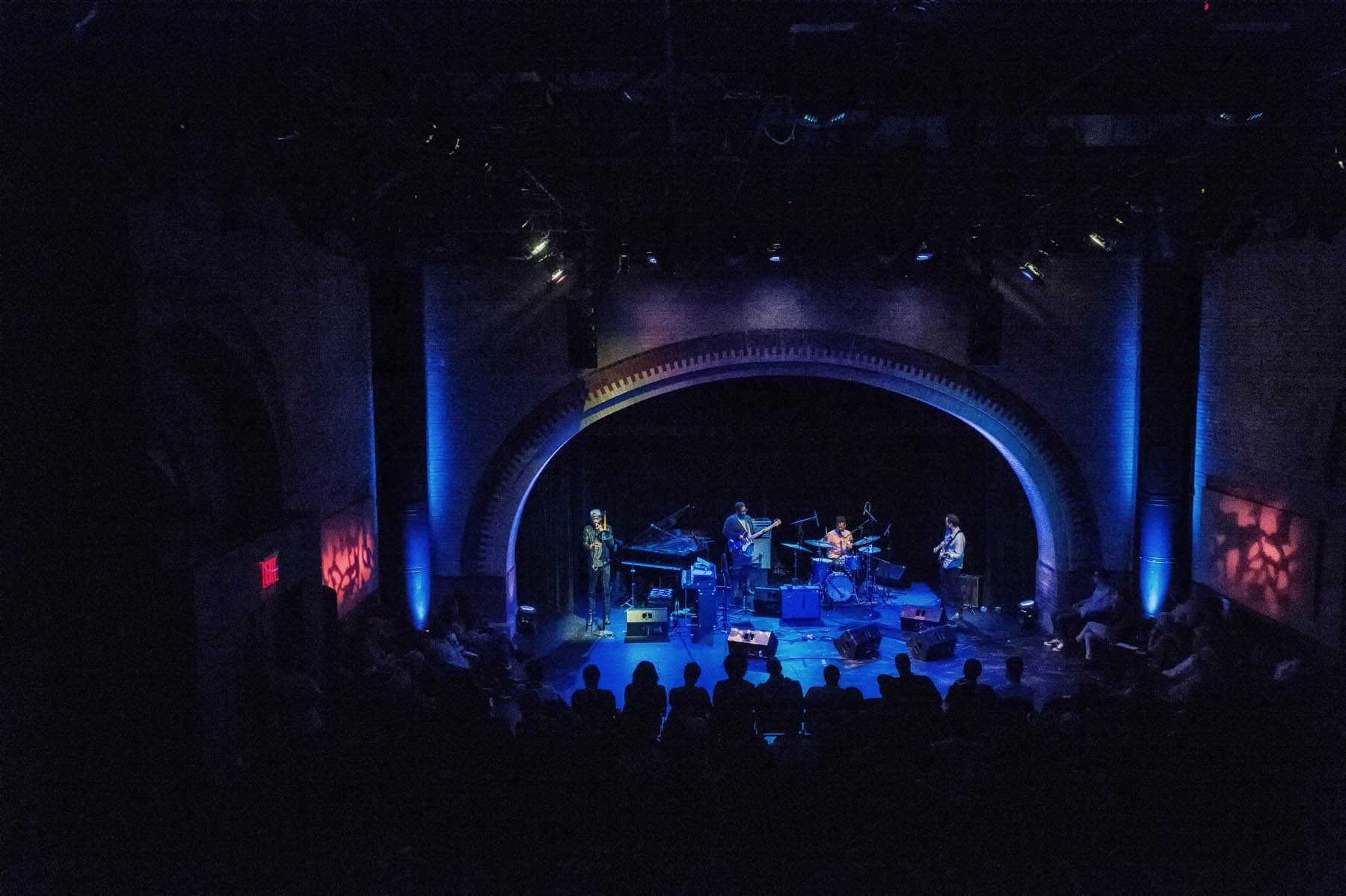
650, 524, 658, 529
863, 503, 867, 515
841, 531, 846, 538
840, 593, 844, 598
814, 512, 819, 527
884, 524, 891, 536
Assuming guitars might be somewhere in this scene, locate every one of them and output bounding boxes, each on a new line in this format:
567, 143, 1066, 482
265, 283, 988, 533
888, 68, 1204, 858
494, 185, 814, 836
937, 536, 952, 562
727, 518, 782, 557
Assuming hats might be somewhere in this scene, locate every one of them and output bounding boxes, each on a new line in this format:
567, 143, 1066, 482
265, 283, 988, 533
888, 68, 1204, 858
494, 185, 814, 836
735, 502, 745, 508
590, 509, 603, 517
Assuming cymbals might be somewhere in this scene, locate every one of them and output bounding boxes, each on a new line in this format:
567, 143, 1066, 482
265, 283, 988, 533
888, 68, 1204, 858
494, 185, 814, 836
804, 540, 835, 548
781, 543, 813, 553
858, 546, 882, 553
853, 535, 881, 546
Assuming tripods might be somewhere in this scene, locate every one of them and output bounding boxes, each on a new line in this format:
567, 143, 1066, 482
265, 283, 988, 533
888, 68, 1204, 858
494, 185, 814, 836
859, 528, 905, 613
658, 529, 695, 638
718, 553, 762, 623
621, 568, 640, 609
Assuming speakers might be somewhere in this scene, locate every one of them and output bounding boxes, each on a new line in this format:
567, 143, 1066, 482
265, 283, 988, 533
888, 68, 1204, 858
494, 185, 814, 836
727, 627, 779, 658
959, 575, 985, 608
900, 604, 957, 662
746, 536, 821, 619
834, 623, 882, 660
874, 561, 912, 588
626, 606, 669, 637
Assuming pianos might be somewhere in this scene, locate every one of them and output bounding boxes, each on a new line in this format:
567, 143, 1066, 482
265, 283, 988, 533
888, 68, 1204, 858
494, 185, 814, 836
621, 501, 716, 616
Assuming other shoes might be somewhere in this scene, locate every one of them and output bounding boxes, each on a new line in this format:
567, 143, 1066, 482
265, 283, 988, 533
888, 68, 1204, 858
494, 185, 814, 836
949, 613, 963, 620
1044, 638, 1059, 646
604, 614, 610, 624
587, 615, 593, 625
747, 588, 754, 595
1083, 659, 1092, 669
1052, 643, 1064, 651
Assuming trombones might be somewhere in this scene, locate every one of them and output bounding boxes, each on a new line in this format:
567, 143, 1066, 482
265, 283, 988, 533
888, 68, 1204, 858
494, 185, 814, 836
591, 510, 614, 572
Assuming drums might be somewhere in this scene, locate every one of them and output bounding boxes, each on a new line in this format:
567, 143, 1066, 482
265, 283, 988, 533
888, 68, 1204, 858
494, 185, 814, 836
823, 572, 854, 602
844, 556, 863, 570
812, 558, 833, 583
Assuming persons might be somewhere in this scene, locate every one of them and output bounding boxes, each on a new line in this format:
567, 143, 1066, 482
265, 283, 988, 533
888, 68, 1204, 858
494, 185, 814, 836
882, 653, 943, 711
804, 665, 850, 705
944, 659, 996, 712
756, 657, 803, 704
571, 665, 615, 712
584, 509, 615, 626
275, 563, 520, 693
625, 661, 667, 719
934, 514, 966, 621
824, 516, 853, 561
723, 502, 763, 595
514, 659, 568, 712
1051, 567, 1315, 705
995, 657, 1033, 706
713, 654, 756, 705
669, 662, 713, 709
